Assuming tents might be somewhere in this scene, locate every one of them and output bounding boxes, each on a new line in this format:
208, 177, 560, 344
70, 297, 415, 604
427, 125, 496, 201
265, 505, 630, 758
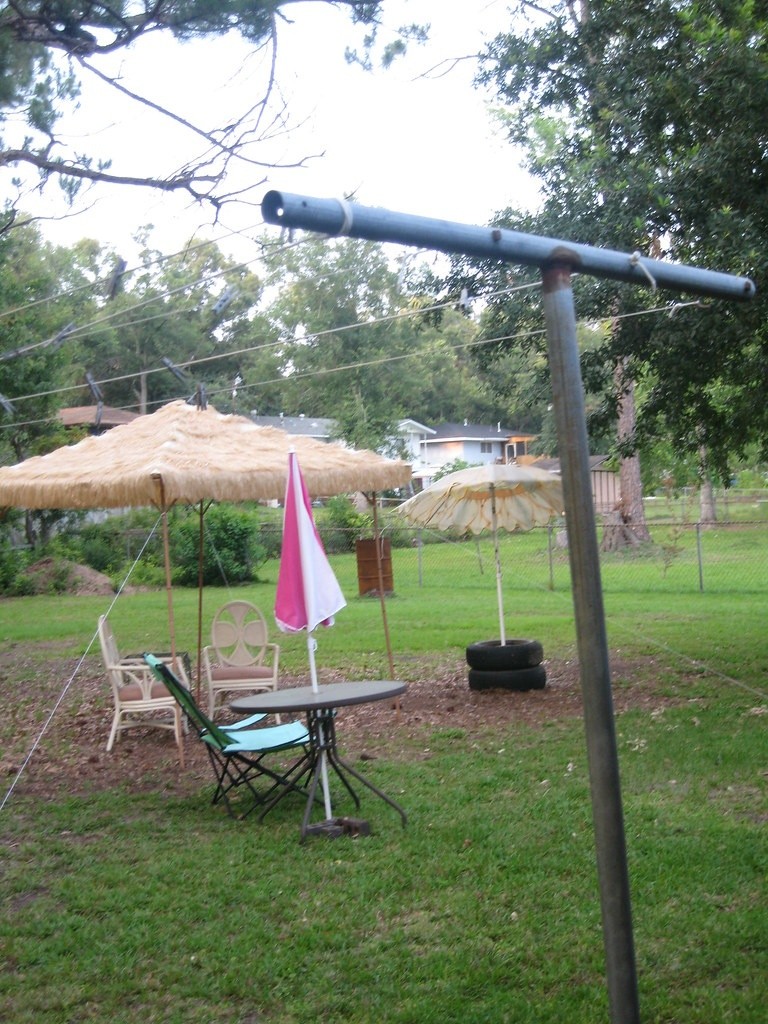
1, 398, 412, 771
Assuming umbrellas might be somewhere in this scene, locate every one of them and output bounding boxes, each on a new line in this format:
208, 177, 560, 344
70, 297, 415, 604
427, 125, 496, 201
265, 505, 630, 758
386, 462, 565, 645
271, 445, 348, 820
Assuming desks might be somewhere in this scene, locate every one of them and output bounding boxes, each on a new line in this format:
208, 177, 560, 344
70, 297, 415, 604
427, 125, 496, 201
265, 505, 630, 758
229, 682, 409, 844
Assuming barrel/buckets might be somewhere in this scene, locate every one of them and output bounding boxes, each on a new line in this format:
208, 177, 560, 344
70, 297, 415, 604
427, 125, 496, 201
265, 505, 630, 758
356, 537, 394, 595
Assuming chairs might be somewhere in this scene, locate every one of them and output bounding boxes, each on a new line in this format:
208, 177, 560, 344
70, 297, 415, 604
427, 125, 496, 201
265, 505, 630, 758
203, 601, 282, 726
97, 614, 189, 751
143, 652, 333, 819
